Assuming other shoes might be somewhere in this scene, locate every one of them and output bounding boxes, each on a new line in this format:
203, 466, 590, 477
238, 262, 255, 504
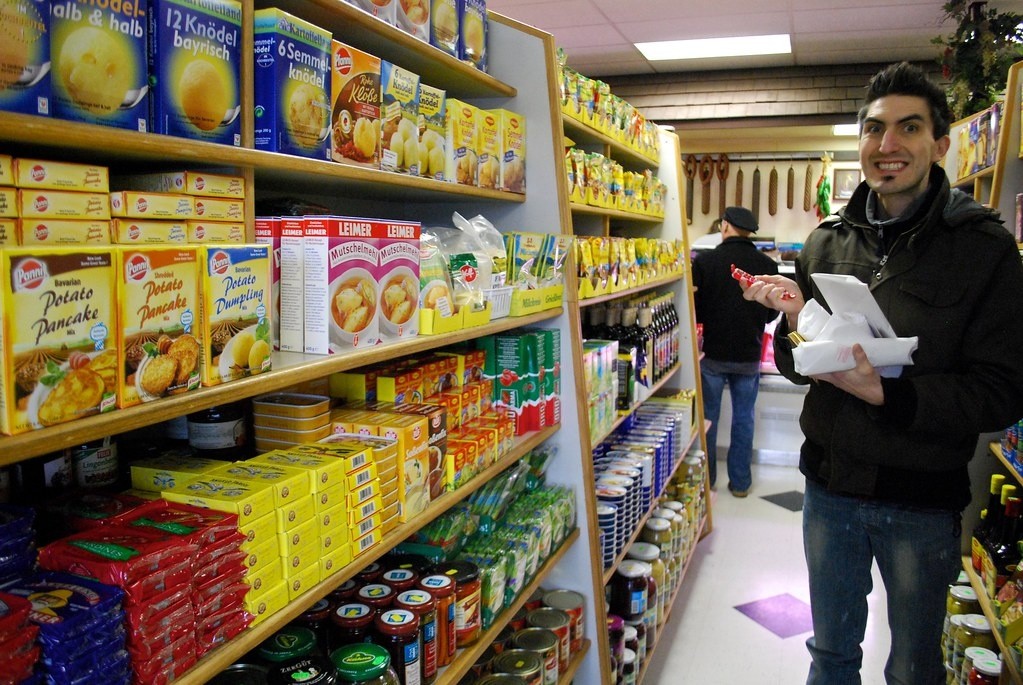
728, 480, 747, 497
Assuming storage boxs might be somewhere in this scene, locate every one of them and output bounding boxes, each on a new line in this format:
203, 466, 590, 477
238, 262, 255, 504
581, 337, 620, 447
1, 1, 565, 683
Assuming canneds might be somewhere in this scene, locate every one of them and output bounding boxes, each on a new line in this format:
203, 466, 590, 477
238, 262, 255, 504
203, 550, 586, 685
593, 413, 706, 685
940, 570, 1003, 685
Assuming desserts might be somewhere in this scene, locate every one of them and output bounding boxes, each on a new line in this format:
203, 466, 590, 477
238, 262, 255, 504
38, 334, 202, 426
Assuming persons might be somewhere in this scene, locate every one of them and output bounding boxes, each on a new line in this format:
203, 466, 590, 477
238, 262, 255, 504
740, 64, 1023, 685
692, 206, 779, 496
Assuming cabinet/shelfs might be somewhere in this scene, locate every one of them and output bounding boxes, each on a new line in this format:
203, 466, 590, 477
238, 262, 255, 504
480, 9, 713, 685
935, 64, 1023, 684
0, 0, 602, 685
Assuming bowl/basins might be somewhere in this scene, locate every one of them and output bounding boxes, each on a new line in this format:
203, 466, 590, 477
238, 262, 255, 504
330, 266, 380, 345
380, 266, 419, 337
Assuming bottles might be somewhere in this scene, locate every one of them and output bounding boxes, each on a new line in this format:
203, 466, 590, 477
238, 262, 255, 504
971, 474, 1022, 599
580, 292, 679, 390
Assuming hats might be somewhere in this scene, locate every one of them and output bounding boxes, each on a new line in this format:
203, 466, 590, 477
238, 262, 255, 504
724, 207, 758, 232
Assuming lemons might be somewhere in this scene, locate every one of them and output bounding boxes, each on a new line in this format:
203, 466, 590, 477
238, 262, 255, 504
391, 119, 446, 176
230, 333, 269, 375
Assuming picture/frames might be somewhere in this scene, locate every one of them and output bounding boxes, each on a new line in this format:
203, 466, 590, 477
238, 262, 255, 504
833, 168, 861, 200
